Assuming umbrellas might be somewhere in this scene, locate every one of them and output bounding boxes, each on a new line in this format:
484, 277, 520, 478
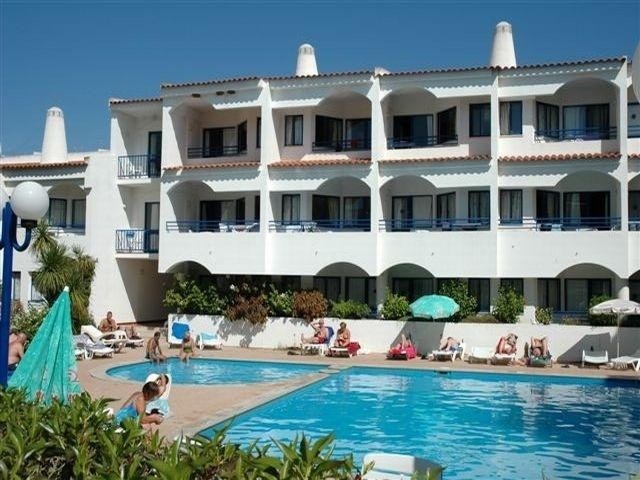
6, 285, 82, 409
409, 293, 460, 322
588, 297, 640, 358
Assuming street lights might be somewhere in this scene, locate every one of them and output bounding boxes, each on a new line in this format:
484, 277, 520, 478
0, 181, 50, 395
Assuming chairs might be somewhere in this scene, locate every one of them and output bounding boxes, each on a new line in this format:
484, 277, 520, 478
142, 374, 171, 416
300, 327, 361, 358
168, 321, 223, 351
385, 328, 555, 367
607, 349, 640, 372
73, 324, 144, 360
361, 453, 413, 480
581, 346, 609, 368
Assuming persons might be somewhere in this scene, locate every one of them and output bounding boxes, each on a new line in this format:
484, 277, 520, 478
146, 372, 169, 405
334, 322, 350, 347
301, 319, 328, 344
146, 331, 166, 366
112, 381, 165, 445
496, 333, 518, 355
441, 336, 460, 352
8, 327, 28, 370
531, 336, 547, 359
392, 334, 412, 350
100, 311, 116, 340
176, 332, 195, 366
